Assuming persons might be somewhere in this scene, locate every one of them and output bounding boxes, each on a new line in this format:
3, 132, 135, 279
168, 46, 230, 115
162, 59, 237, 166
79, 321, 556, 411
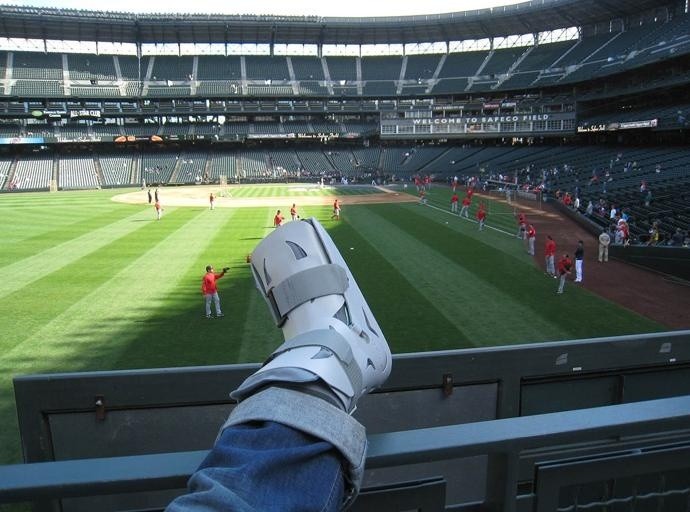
140, 151, 399, 228
413, 150, 685, 294
161, 219, 392, 512
200, 264, 231, 320
